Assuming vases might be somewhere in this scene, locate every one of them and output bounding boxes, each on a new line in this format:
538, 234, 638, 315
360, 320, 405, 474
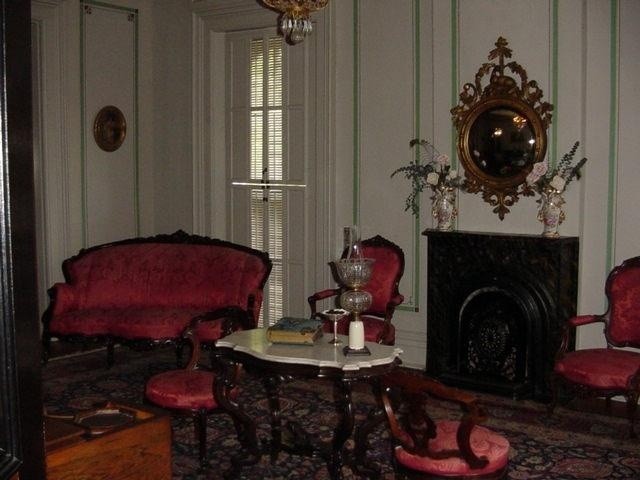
435, 188, 454, 231
538, 188, 565, 239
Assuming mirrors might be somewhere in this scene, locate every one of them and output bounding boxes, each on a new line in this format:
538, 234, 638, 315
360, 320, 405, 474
450, 35, 554, 220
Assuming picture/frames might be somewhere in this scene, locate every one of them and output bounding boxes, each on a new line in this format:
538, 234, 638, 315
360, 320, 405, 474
93, 106, 127, 152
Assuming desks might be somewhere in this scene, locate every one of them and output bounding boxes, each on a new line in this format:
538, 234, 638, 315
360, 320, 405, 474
212, 325, 405, 480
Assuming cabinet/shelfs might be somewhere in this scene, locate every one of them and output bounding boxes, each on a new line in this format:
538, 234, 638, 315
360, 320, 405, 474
421, 229, 578, 402
42, 395, 173, 479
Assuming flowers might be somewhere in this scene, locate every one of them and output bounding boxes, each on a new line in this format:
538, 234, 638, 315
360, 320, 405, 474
526, 141, 588, 200
390, 140, 469, 217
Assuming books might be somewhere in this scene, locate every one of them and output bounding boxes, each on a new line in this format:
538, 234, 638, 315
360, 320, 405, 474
266, 317, 326, 346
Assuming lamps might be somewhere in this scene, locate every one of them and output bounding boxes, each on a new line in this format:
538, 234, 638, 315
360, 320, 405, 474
259, 0, 331, 44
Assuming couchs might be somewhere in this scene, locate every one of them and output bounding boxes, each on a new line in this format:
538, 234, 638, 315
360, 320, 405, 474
41, 228, 273, 368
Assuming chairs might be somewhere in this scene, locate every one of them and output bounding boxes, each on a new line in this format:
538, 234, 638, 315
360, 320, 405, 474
546, 257, 640, 425
372, 376, 510, 480
308, 238, 405, 346
146, 307, 249, 468
